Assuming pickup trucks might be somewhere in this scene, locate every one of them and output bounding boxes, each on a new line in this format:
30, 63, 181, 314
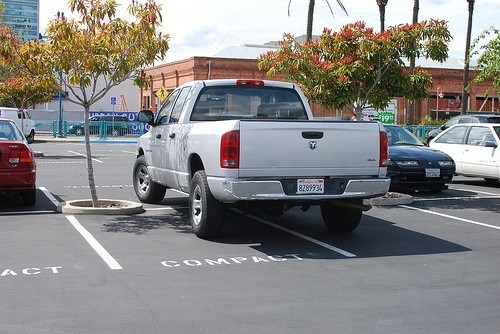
132, 78, 391, 239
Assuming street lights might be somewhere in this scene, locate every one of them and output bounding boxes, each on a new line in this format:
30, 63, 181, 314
37, 12, 64, 138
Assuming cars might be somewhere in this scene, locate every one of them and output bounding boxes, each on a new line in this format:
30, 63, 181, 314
0, 118, 38, 207
429, 122, 500, 184
427, 114, 499, 143
381, 123, 455, 193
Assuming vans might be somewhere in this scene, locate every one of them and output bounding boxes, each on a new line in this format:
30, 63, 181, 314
69, 115, 131, 136
0, 106, 36, 144
